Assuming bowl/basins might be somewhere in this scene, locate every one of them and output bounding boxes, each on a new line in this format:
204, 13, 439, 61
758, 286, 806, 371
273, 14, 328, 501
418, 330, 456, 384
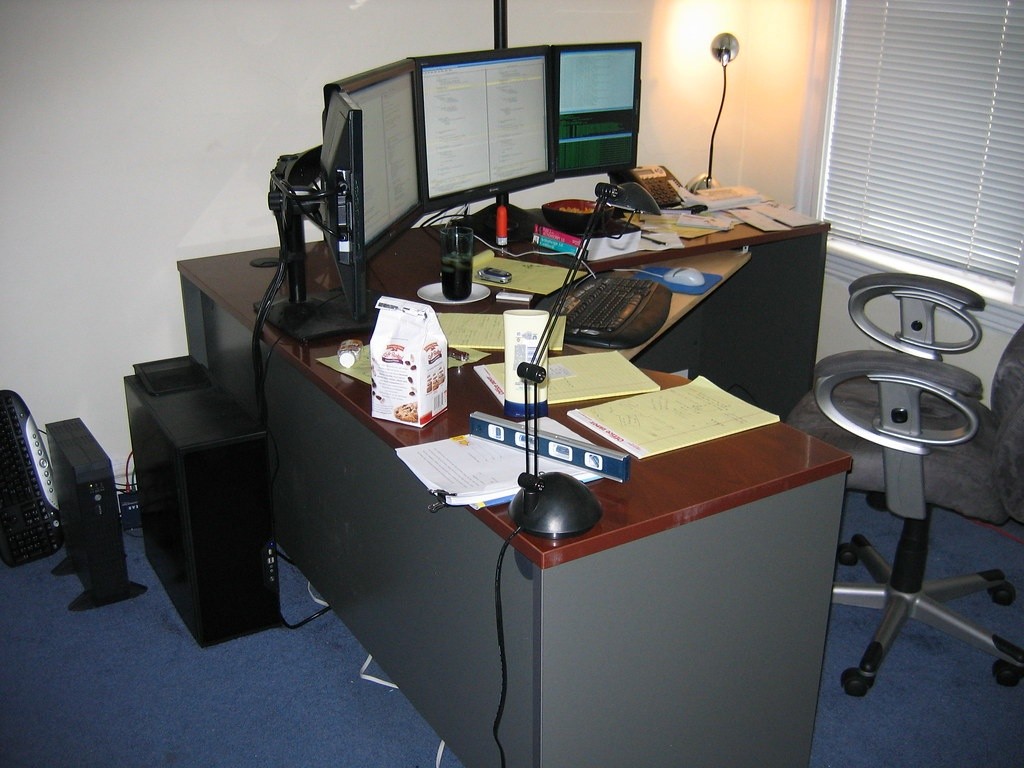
542, 199, 615, 233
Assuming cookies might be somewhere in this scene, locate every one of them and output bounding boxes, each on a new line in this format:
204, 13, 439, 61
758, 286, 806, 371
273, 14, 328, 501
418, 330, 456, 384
395, 402, 418, 423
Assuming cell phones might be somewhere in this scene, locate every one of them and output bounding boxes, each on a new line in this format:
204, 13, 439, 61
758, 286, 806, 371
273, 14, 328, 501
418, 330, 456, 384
475, 266, 512, 284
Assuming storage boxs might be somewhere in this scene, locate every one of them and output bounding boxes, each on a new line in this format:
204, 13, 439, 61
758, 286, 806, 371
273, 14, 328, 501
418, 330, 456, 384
579, 219, 641, 260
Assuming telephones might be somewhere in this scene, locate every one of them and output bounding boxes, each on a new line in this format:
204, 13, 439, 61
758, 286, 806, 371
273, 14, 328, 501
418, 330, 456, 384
607, 165, 684, 210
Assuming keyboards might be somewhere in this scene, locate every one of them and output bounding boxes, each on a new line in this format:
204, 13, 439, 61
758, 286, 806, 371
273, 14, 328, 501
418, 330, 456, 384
536, 272, 673, 349
0, 390, 64, 567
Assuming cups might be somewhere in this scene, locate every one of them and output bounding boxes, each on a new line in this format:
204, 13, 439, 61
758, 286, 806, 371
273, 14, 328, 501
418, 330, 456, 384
440, 226, 473, 302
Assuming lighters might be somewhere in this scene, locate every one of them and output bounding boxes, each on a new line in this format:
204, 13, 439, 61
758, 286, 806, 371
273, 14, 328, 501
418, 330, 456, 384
495, 292, 533, 301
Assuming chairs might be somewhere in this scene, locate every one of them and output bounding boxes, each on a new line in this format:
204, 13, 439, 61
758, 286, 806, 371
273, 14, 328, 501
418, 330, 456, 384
785, 272, 1023, 698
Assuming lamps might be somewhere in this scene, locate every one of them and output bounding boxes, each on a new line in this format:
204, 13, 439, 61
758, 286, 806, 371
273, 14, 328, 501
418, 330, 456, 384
509, 183, 662, 544
686, 33, 739, 195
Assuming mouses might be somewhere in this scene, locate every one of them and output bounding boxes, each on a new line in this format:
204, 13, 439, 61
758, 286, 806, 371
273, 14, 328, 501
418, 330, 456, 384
664, 267, 705, 286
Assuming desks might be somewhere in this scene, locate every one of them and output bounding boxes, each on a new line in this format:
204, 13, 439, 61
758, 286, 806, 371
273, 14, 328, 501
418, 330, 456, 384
176, 204, 855, 768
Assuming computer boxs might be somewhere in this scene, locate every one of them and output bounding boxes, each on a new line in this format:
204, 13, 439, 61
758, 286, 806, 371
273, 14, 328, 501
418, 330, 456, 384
124, 364, 283, 649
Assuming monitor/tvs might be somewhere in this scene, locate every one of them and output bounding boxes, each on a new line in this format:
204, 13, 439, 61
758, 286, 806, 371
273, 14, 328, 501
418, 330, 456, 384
253, 41, 644, 350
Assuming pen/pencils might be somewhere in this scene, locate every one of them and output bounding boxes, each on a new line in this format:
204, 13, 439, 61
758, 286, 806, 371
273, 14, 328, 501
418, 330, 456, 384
640, 235, 666, 247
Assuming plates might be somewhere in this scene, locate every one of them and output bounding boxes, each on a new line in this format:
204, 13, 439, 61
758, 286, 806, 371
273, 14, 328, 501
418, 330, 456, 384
417, 282, 491, 304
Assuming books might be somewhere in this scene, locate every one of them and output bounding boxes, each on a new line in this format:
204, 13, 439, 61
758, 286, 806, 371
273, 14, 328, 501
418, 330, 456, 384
677, 213, 732, 230
696, 188, 760, 211
532, 221, 642, 261
